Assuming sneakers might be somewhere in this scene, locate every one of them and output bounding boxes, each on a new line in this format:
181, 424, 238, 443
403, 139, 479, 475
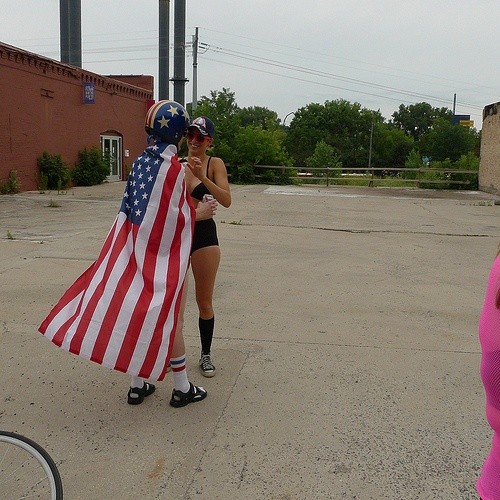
199, 353, 216, 377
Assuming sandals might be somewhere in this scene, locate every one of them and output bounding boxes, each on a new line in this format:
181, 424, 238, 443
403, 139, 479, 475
169, 382, 208, 408
126, 381, 156, 405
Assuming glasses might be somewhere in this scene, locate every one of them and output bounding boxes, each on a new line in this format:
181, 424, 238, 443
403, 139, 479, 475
185, 133, 211, 142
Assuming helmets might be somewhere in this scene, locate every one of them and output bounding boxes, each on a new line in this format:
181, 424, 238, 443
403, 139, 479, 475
144, 99, 190, 141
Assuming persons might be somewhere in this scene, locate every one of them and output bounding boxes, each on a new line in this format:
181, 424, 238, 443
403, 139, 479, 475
477, 242, 500, 500
166, 116, 231, 378
36, 100, 219, 408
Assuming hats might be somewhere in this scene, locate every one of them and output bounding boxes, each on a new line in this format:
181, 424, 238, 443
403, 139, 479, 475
189, 116, 215, 138
